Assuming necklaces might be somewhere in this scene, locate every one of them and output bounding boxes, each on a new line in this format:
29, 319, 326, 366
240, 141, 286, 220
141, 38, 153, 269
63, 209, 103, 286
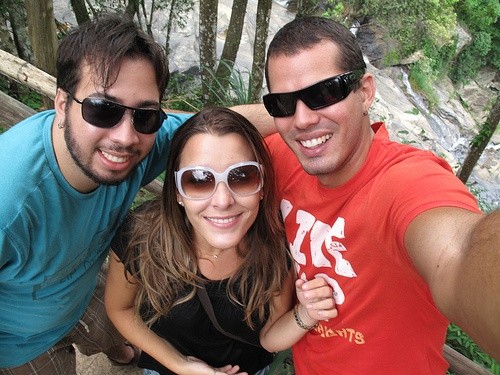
213, 254, 218, 258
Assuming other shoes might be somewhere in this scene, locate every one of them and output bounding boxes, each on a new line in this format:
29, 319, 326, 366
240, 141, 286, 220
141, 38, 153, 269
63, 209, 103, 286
107, 344, 140, 365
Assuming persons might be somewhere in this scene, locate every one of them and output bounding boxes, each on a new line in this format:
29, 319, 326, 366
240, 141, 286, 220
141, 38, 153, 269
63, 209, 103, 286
0, 13, 375, 375
104, 106, 338, 375
263, 16, 500, 375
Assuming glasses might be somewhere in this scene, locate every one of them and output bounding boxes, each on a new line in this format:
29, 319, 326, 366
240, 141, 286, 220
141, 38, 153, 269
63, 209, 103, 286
175, 161, 264, 200
65, 89, 167, 136
263, 69, 365, 117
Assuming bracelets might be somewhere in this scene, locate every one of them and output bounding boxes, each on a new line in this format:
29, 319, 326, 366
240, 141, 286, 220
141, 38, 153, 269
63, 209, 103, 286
293, 304, 319, 329
